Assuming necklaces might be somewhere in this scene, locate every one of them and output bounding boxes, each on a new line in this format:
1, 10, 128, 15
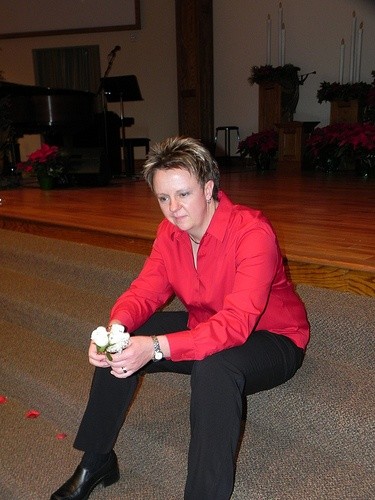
189, 199, 216, 244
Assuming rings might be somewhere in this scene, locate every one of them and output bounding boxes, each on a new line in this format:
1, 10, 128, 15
122, 367, 127, 373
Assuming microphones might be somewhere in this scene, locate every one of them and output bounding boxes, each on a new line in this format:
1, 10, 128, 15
109, 45, 120, 56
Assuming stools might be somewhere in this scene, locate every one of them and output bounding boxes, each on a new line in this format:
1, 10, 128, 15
214, 126, 240, 160
119, 138, 151, 176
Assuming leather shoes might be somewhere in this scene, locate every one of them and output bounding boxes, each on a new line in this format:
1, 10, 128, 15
49, 449, 121, 500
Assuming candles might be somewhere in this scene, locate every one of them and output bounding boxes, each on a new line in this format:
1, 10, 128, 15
339, 11, 364, 84
266, 1, 285, 67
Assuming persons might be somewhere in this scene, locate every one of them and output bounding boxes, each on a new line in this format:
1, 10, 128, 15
49, 136, 310, 500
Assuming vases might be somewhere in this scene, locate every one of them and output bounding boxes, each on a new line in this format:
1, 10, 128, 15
355, 152, 370, 176
255, 152, 276, 170
37, 174, 58, 189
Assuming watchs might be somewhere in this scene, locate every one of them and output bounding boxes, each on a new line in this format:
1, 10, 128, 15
152, 335, 163, 362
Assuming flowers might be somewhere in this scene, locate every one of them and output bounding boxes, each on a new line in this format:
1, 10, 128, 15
235, 128, 279, 158
307, 120, 375, 167
90, 324, 131, 362
14, 144, 64, 177
